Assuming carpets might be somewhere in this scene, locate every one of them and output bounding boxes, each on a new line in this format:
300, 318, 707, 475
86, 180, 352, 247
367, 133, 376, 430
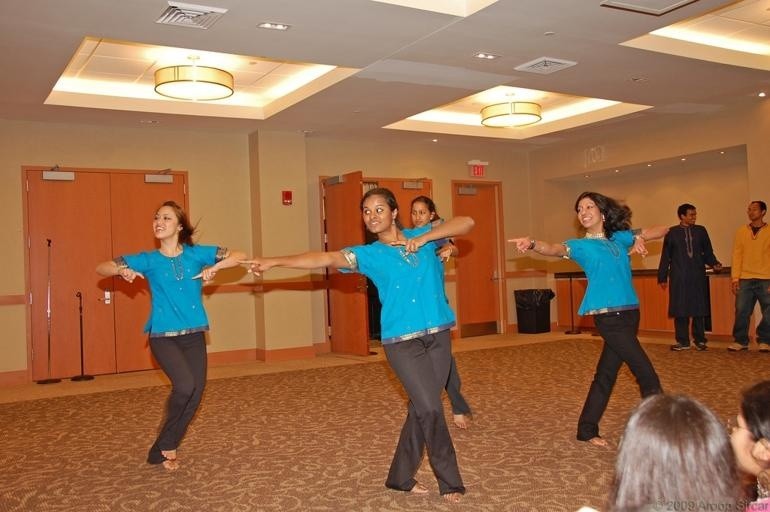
0, 339, 770, 512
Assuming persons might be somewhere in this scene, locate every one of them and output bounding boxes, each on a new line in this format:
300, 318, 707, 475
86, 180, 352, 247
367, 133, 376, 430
656, 203, 723, 352
410, 196, 472, 430
237, 188, 476, 504
94, 199, 248, 472
578, 391, 749, 512
729, 378, 770, 512
507, 192, 670, 446
726, 200, 769, 353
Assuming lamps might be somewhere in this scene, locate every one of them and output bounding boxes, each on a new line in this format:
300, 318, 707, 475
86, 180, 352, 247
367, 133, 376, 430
481, 91, 542, 127
155, 55, 234, 101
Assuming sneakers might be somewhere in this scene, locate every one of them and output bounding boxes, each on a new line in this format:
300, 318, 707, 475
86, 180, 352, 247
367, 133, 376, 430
696, 343, 709, 351
759, 342, 770, 351
672, 342, 691, 350
728, 342, 749, 351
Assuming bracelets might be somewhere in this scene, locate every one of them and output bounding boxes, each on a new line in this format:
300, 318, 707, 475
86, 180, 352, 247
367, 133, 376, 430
528, 236, 537, 251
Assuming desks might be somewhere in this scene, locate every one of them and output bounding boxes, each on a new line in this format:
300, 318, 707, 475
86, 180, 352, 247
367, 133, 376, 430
555, 267, 736, 335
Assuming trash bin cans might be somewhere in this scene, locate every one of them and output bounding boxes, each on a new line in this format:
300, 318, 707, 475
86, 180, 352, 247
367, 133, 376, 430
513, 288, 551, 334
